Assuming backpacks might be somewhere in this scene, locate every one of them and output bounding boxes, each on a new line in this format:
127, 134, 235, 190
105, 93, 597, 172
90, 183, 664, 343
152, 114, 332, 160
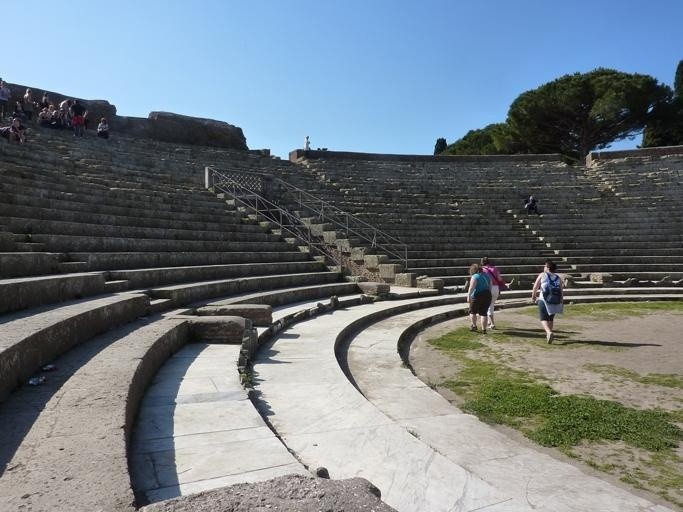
542, 273, 562, 304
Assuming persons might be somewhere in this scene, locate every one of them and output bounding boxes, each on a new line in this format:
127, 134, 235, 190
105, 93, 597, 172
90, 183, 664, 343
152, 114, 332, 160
303, 136, 311, 152
96, 117, 109, 138
530, 259, 564, 344
477, 256, 515, 329
522, 194, 540, 215
466, 262, 493, 334
0, 78, 90, 145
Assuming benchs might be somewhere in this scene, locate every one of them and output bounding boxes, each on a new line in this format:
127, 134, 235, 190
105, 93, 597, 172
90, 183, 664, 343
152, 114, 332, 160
329, 200, 682, 287
0, 173, 396, 511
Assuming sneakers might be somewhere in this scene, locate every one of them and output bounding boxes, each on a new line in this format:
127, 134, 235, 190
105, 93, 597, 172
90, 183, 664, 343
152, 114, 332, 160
469, 321, 495, 334
546, 332, 552, 344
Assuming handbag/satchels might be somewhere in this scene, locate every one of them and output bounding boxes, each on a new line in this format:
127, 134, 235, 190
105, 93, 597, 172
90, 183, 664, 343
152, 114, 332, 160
497, 280, 505, 289
